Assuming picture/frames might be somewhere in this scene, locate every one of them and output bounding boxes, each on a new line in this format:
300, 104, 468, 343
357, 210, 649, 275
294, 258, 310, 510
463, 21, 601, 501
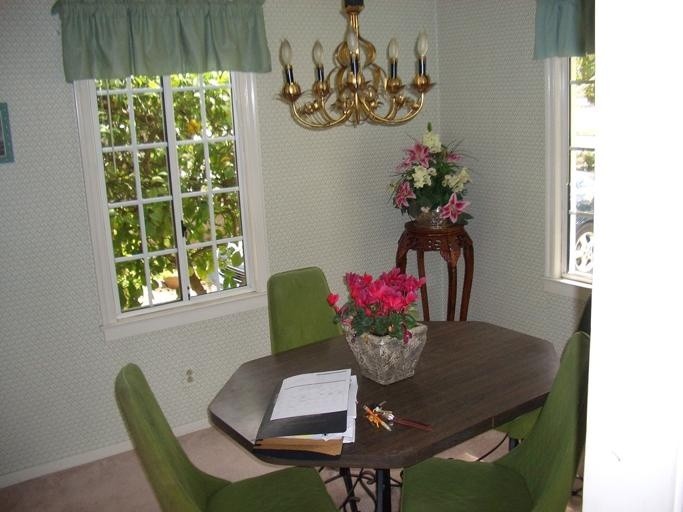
0, 103, 14, 165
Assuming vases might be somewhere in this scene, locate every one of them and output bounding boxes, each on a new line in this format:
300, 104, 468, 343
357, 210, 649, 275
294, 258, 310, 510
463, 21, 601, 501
415, 209, 450, 228
338, 315, 427, 383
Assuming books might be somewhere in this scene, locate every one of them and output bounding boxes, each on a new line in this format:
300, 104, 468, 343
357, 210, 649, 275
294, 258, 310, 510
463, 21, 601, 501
254, 365, 360, 457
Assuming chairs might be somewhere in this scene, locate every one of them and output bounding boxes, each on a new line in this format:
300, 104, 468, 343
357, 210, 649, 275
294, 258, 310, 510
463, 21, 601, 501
400, 331, 588, 511
116, 359, 337, 511
496, 294, 590, 455
265, 264, 344, 358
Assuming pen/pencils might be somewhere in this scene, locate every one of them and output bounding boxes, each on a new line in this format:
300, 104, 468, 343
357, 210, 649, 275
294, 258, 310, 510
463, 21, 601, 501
363, 405, 391, 431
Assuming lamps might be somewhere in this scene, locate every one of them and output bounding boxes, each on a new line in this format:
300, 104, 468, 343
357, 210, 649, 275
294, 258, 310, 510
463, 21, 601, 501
274, 0, 436, 130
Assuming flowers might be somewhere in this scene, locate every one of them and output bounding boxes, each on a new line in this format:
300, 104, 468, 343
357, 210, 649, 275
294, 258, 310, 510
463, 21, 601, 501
389, 121, 472, 224
328, 265, 430, 341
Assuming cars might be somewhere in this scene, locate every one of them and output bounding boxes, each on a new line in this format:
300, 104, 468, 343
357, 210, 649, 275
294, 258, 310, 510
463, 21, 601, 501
205, 235, 246, 290
568, 171, 595, 281
